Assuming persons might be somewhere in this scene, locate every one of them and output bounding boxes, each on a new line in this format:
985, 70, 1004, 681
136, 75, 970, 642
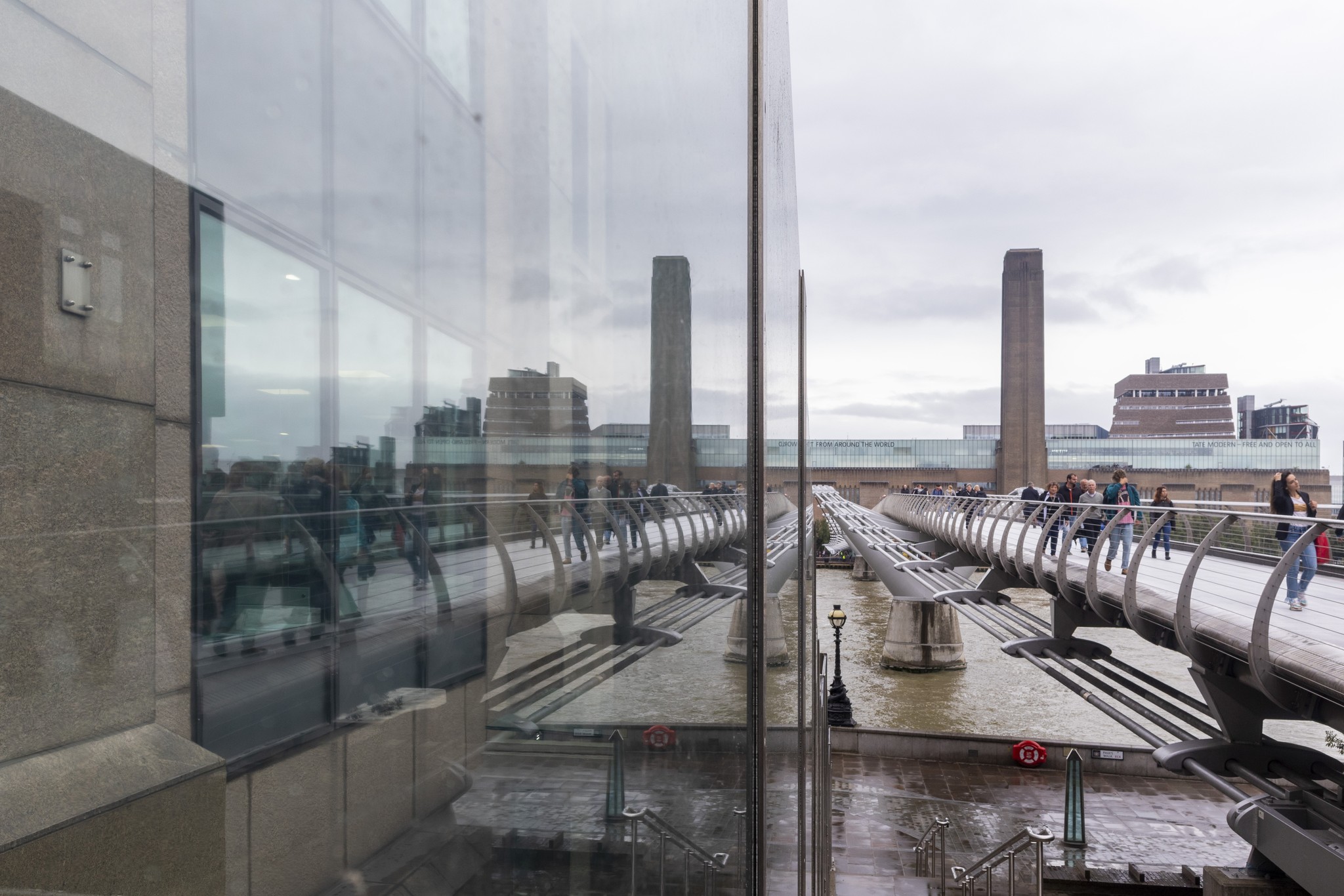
822, 550, 825, 557
553, 465, 650, 565
766, 483, 772, 494
1269, 470, 1317, 612
702, 481, 746, 527
1078, 479, 1089, 553
931, 484, 945, 517
901, 484, 910, 494
956, 486, 964, 514
977, 486, 988, 520
944, 485, 957, 519
816, 550, 821, 557
1034, 481, 1069, 558
910, 484, 931, 517
525, 481, 549, 548
1335, 503, 1344, 543
880, 495, 886, 501
1077, 479, 1104, 558
1103, 469, 1143, 575
973, 485, 980, 511
958, 484, 977, 530
190, 456, 445, 659
1021, 481, 1040, 528
1149, 487, 1177, 560
650, 479, 669, 523
959, 483, 967, 496
1057, 473, 1079, 554
837, 551, 848, 556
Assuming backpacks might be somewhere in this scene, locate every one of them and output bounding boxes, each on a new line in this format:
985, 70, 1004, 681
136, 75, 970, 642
912, 489, 918, 494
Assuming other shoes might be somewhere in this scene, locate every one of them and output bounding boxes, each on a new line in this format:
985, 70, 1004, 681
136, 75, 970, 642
1104, 560, 1111, 571
1122, 569, 1128, 575
581, 549, 587, 561
1081, 547, 1086, 552
596, 544, 603, 551
653, 520, 665, 523
413, 573, 425, 591
563, 559, 572, 564
604, 538, 611, 544
1298, 595, 1307, 606
1289, 603, 1302, 611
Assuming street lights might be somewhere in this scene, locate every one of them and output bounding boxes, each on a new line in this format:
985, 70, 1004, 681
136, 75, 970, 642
826, 602, 860, 727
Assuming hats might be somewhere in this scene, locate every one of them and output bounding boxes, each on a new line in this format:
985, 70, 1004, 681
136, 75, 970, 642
1027, 482, 1035, 487
963, 483, 967, 486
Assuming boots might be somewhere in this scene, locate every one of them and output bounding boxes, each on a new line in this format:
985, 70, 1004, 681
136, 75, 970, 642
1151, 549, 1156, 558
542, 538, 547, 547
529, 540, 535, 548
1166, 552, 1171, 560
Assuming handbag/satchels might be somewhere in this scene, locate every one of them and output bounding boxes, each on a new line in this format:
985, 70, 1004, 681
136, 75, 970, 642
1314, 532, 1330, 563
952, 494, 956, 499
391, 525, 405, 548
